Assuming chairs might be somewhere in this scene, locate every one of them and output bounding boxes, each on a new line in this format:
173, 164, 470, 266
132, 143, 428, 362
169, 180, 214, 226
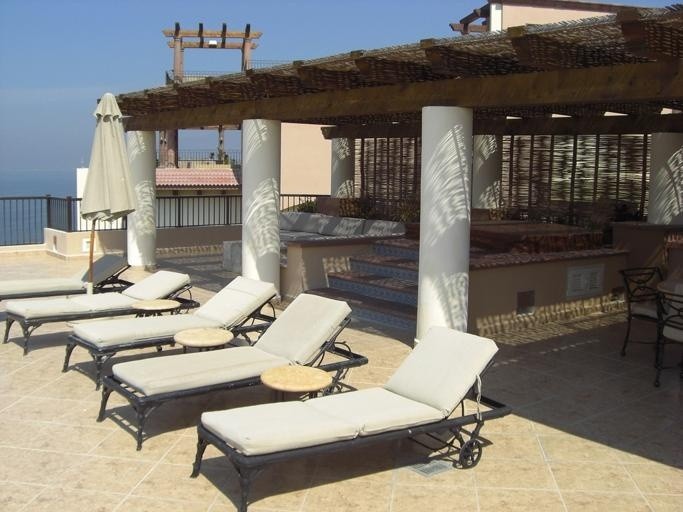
619, 266, 663, 368
654, 292, 683, 387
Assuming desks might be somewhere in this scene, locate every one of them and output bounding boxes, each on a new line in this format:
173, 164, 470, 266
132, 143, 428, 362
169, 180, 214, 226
657, 279, 683, 294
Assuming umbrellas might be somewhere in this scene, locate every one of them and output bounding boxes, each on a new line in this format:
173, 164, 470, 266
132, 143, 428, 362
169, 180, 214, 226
80, 92, 134, 294
